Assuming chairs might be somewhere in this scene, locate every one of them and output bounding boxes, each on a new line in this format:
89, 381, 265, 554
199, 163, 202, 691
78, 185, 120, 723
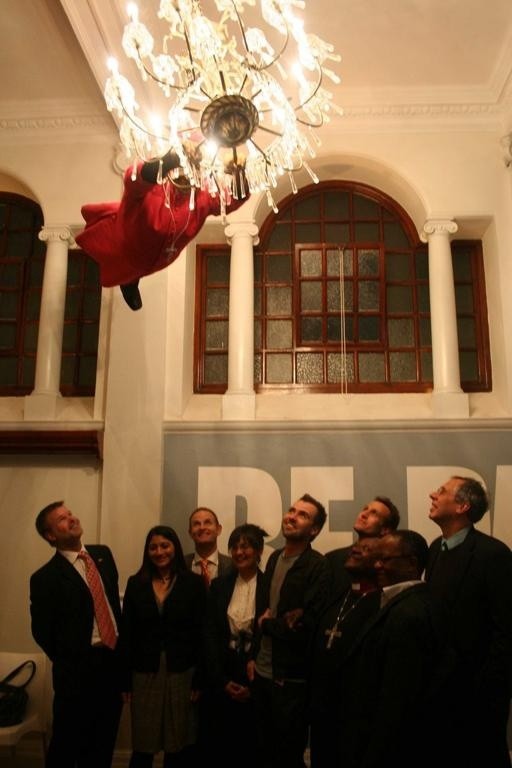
0, 650, 51, 768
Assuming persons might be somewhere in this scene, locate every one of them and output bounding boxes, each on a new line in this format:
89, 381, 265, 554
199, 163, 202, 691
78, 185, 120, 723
312, 537, 382, 768
264, 495, 326, 767
324, 496, 403, 591
181, 508, 233, 586
119, 525, 215, 768
352, 532, 454, 768
424, 475, 512, 742
30, 502, 122, 768
77, 146, 250, 311
201, 524, 268, 768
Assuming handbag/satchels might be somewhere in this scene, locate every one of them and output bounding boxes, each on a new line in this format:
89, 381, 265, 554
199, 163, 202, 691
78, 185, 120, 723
1, 660, 37, 726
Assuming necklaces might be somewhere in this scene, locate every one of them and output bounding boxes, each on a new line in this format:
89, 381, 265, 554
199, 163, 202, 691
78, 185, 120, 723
328, 581, 368, 647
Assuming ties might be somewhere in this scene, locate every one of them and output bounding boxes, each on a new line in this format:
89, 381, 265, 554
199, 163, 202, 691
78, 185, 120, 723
200, 560, 210, 591
77, 551, 117, 650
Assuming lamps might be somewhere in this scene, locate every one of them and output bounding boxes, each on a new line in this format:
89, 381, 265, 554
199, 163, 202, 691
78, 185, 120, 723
105, 1, 339, 223
